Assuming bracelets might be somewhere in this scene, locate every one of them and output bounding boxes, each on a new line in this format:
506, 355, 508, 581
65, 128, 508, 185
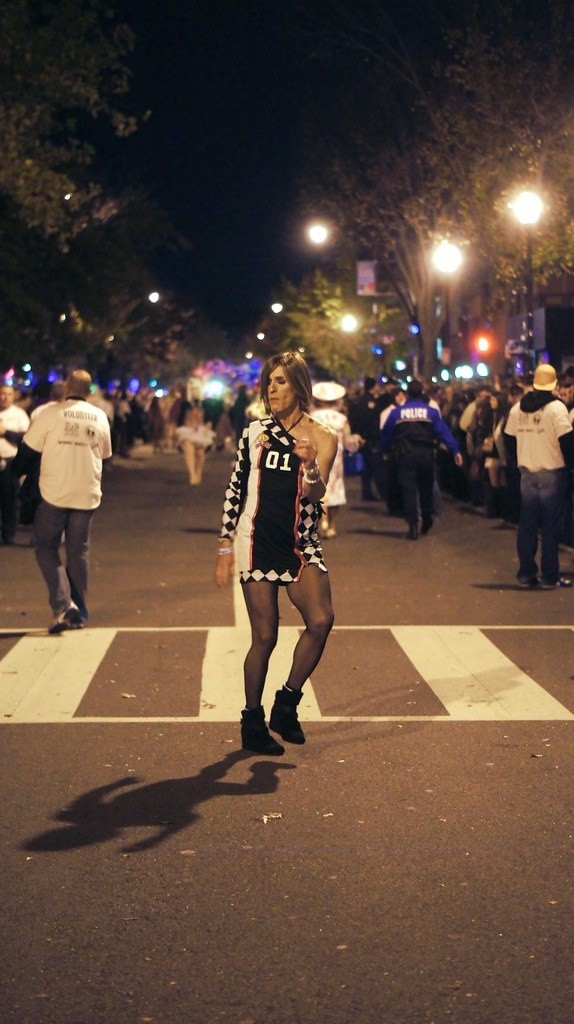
216, 547, 233, 554
299, 462, 322, 483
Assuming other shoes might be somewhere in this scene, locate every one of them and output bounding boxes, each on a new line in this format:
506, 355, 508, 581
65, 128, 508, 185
46, 601, 81, 633
325, 530, 337, 538
541, 574, 574, 588
518, 575, 540, 585
408, 523, 419, 539
67, 606, 90, 629
419, 523, 433, 535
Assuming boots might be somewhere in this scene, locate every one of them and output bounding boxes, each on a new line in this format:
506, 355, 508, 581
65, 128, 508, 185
269, 690, 308, 745
241, 706, 286, 756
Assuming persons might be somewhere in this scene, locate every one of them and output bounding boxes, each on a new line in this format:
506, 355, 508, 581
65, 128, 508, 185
215, 350, 339, 754
0, 364, 572, 548
499, 365, 574, 588
10, 370, 112, 633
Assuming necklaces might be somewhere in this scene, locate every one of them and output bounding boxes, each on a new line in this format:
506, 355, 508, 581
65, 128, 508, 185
286, 414, 305, 434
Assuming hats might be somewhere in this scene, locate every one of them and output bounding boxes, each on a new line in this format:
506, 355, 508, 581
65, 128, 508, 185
311, 382, 347, 403
533, 363, 558, 391
407, 380, 423, 399
364, 378, 375, 391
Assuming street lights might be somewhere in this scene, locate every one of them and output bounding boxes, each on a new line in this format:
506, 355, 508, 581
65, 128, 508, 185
310, 224, 424, 366
515, 193, 544, 385
433, 243, 464, 362
